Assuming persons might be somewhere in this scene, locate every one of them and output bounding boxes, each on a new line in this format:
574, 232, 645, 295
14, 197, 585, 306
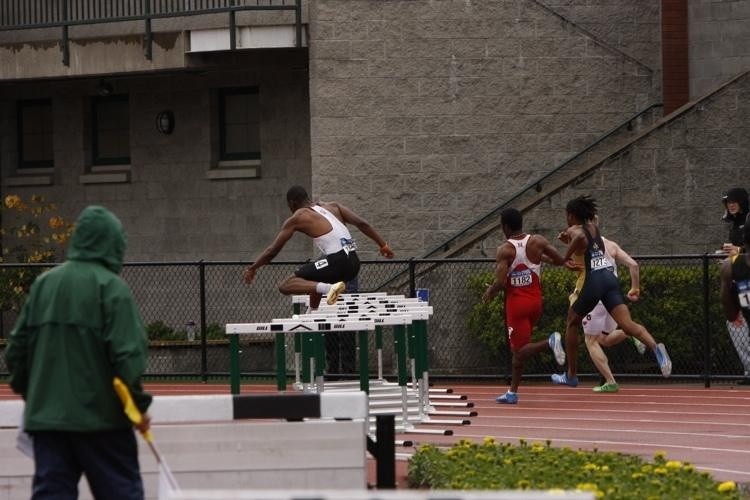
720, 214, 750, 380
482, 208, 566, 405
556, 214, 647, 394
712, 187, 750, 387
239, 183, 394, 315
548, 193, 673, 387
4, 205, 154, 499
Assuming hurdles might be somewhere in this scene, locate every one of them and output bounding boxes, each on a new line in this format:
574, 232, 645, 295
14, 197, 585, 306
1, 391, 366, 431
224, 292, 478, 461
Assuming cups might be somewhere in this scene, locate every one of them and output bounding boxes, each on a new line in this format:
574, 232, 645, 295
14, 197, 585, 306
185, 320, 196, 342
722, 241, 733, 254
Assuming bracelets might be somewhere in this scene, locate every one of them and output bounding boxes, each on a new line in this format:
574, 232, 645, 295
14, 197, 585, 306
631, 287, 640, 293
380, 243, 389, 252
246, 265, 255, 272
736, 245, 741, 253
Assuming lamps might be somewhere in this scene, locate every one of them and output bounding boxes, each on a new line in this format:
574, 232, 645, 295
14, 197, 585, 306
95, 79, 114, 97
156, 111, 175, 135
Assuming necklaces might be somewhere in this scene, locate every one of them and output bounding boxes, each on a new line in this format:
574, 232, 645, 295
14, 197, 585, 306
506, 233, 527, 240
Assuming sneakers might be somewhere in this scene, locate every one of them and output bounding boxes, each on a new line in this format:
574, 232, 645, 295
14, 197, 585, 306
327, 281, 346, 305
632, 337, 646, 355
495, 392, 518, 404
548, 331, 567, 366
551, 371, 578, 387
655, 343, 673, 378
594, 382, 619, 393
